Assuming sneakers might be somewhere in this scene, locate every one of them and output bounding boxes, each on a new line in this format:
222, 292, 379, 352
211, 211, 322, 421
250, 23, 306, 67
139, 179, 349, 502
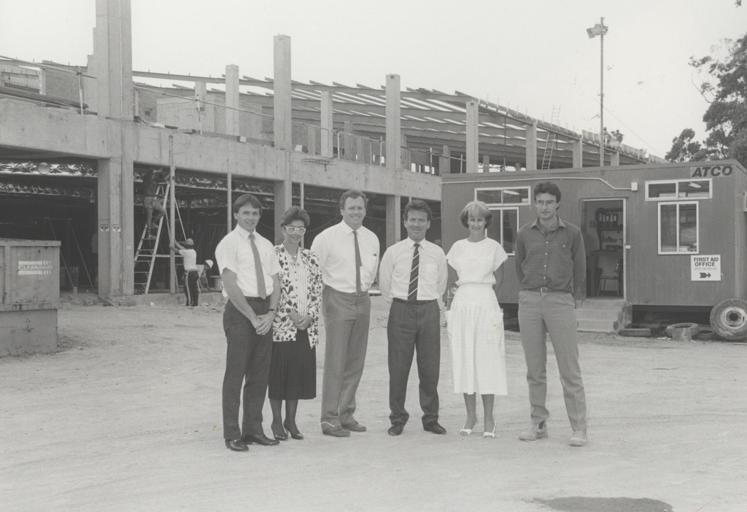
569, 432, 587, 446
520, 423, 547, 441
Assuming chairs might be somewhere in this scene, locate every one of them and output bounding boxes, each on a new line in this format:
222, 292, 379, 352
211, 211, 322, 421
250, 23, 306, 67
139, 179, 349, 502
597, 265, 621, 297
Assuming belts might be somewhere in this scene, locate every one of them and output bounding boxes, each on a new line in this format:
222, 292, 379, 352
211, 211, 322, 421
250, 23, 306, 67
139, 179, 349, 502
528, 286, 554, 293
394, 298, 433, 304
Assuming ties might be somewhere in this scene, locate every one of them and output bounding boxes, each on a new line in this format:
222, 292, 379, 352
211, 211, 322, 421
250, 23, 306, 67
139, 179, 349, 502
249, 233, 266, 300
353, 232, 362, 295
409, 244, 419, 304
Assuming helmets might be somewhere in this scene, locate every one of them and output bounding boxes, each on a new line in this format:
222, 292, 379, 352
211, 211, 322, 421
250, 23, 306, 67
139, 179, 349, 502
184, 239, 193, 245
206, 260, 213, 268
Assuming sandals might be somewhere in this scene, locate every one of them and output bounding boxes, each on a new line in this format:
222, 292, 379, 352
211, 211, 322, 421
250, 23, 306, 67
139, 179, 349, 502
483, 421, 496, 440
460, 423, 476, 436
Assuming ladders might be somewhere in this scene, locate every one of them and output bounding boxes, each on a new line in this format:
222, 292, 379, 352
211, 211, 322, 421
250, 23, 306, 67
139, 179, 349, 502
134, 182, 187, 295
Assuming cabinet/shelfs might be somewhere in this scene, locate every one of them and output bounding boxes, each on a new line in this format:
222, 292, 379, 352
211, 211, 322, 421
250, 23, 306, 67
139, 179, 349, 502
595, 208, 623, 251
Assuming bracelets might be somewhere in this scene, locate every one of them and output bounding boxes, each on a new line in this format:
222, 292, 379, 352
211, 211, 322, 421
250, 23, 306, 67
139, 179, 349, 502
268, 308, 277, 314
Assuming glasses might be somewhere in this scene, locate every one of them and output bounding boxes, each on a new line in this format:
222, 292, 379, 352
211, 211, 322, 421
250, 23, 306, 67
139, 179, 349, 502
284, 225, 306, 236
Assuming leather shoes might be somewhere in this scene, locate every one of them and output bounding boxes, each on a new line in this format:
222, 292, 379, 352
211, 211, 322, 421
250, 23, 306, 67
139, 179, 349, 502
323, 428, 350, 437
388, 423, 404, 435
226, 439, 248, 451
271, 424, 287, 440
284, 420, 303, 439
345, 421, 366, 431
424, 422, 446, 434
242, 432, 279, 446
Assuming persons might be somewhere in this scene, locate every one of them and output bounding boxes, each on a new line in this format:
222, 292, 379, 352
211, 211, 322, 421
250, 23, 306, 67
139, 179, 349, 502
442, 200, 508, 438
168, 238, 198, 306
513, 181, 587, 446
311, 189, 381, 438
380, 199, 448, 435
267, 205, 322, 441
214, 194, 279, 452
195, 259, 213, 292
140, 167, 168, 239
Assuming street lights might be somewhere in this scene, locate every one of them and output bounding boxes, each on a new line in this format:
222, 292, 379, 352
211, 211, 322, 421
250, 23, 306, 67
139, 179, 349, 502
586, 16, 609, 167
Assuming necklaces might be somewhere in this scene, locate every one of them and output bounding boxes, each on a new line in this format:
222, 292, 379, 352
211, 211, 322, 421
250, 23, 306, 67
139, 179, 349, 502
289, 254, 297, 259
